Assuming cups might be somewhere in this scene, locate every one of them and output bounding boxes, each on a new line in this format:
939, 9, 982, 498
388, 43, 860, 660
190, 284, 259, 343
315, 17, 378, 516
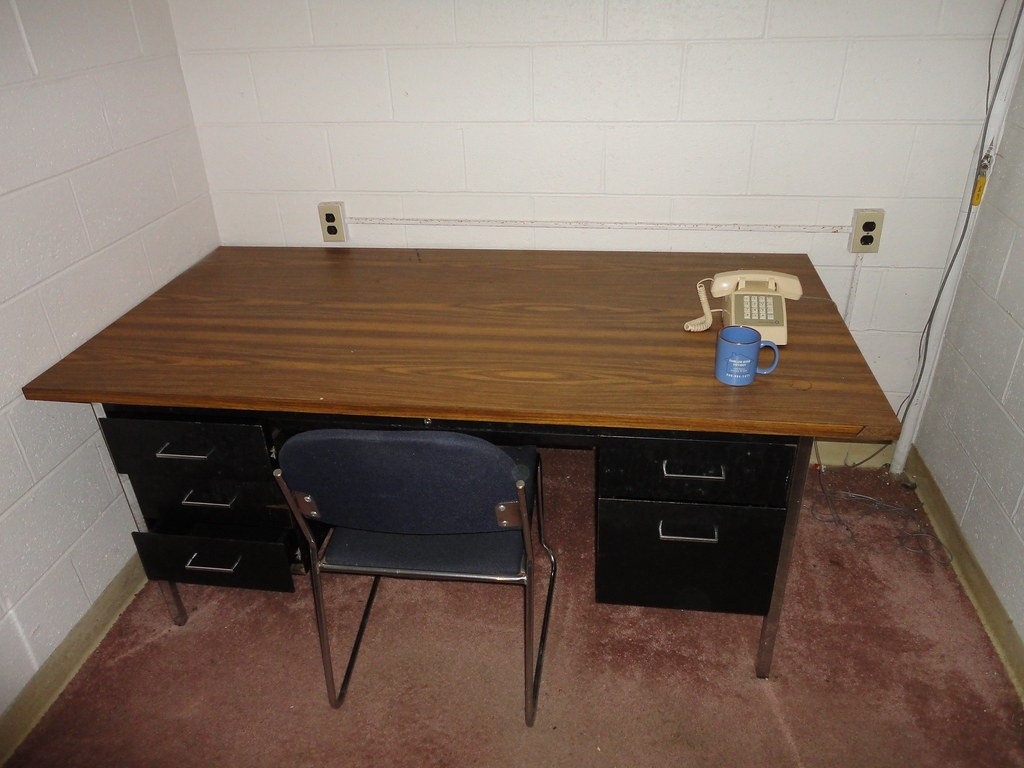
715, 325, 779, 386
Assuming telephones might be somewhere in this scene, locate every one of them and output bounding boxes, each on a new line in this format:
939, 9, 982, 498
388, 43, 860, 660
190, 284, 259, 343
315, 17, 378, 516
710, 269, 804, 348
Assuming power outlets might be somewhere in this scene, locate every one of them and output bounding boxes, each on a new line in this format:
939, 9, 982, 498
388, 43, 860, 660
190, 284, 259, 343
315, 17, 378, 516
318, 201, 346, 242
848, 209, 884, 253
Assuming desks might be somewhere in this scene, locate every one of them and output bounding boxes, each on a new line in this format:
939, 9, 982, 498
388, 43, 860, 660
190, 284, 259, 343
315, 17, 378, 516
21, 245, 903, 679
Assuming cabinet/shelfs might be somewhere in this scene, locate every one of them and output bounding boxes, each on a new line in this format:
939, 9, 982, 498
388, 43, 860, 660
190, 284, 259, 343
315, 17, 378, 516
97, 403, 332, 593
592, 437, 798, 616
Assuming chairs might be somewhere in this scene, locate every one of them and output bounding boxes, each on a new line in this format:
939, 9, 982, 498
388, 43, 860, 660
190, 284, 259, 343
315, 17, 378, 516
274, 430, 557, 727
